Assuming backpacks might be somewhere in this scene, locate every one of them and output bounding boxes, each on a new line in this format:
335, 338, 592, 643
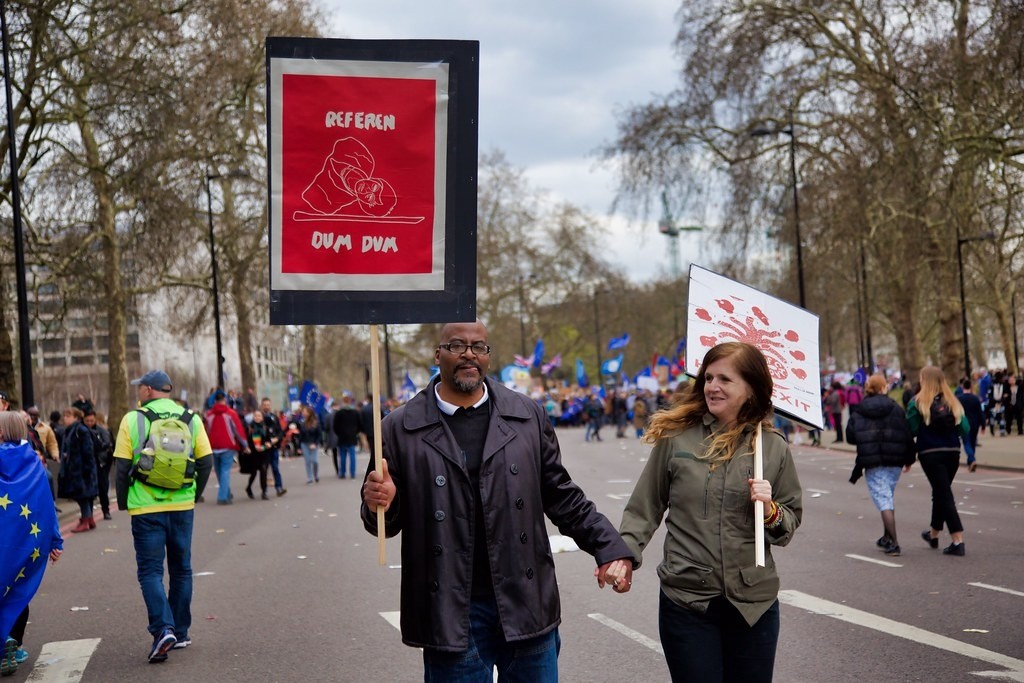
133, 407, 196, 490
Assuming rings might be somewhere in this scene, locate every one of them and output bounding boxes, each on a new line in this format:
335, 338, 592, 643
628, 581, 632, 585
611, 579, 618, 586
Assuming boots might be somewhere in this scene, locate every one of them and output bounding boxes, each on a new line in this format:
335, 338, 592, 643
71, 518, 90, 532
88, 517, 96, 529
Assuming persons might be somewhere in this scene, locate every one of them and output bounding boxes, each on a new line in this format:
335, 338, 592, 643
847, 374, 917, 556
0, 410, 64, 676
112, 370, 214, 663
360, 317, 639, 683
906, 365, 979, 556
593, 342, 802, 683
0, 364, 1024, 535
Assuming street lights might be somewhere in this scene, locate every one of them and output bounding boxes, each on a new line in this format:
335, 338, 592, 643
518, 275, 537, 357
750, 102, 806, 309
593, 288, 610, 384
206, 166, 251, 392
954, 226, 997, 380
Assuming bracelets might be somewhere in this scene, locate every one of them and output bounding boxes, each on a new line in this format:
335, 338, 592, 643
764, 500, 784, 529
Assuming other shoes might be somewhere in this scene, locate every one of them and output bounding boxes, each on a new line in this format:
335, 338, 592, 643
229, 493, 233, 499
104, 514, 111, 519
922, 530, 938, 548
943, 542, 965, 556
0, 634, 18, 678
15, 647, 28, 662
216, 499, 233, 505
246, 487, 255, 499
877, 536, 892, 549
970, 461, 977, 472
262, 493, 270, 500
277, 488, 287, 496
884, 544, 900, 556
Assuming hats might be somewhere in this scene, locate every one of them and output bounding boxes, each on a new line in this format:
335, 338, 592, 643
0, 391, 9, 402
130, 370, 173, 392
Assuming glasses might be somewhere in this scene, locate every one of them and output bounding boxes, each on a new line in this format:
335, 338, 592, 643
439, 342, 490, 355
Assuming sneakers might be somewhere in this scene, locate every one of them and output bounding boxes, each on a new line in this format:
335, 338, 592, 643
173, 636, 192, 648
148, 629, 177, 664
